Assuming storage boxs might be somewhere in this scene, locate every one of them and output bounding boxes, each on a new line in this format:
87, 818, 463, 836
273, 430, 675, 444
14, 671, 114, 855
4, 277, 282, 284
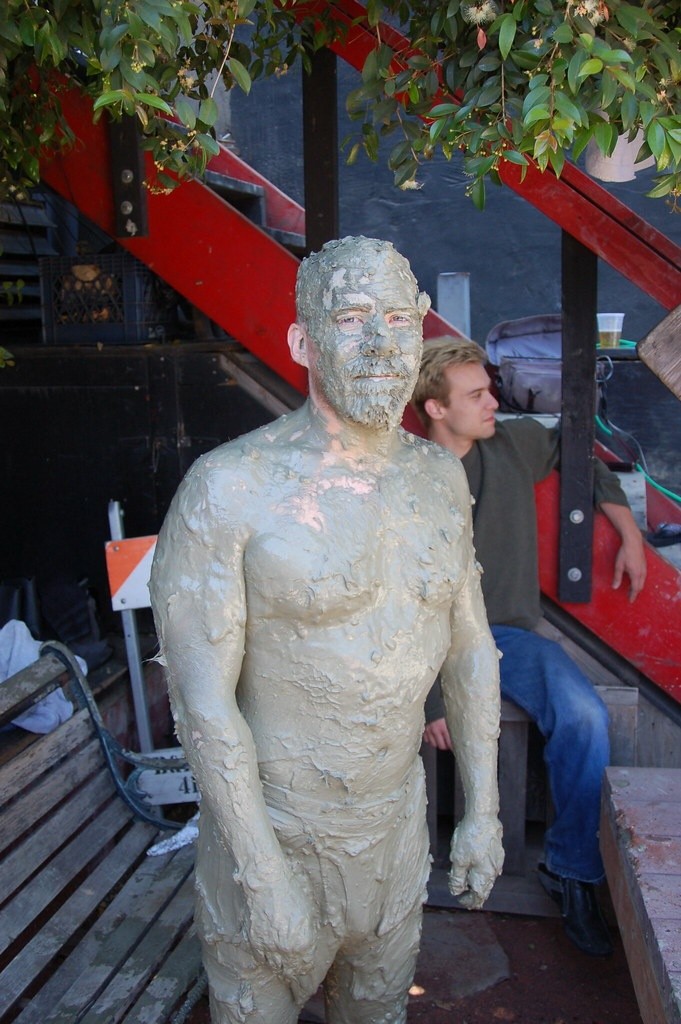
38, 251, 196, 346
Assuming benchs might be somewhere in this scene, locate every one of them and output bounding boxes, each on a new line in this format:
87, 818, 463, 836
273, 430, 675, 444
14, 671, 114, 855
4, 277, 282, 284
0, 639, 208, 1024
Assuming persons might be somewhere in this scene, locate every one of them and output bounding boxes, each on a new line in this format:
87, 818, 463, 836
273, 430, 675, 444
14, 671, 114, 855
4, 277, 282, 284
413, 338, 645, 955
148, 237, 506, 1024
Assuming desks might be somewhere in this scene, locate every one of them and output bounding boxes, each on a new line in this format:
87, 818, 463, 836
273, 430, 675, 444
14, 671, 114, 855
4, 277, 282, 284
595, 339, 681, 503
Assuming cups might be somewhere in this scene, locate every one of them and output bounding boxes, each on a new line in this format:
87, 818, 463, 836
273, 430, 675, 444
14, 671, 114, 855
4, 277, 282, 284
596, 312, 625, 348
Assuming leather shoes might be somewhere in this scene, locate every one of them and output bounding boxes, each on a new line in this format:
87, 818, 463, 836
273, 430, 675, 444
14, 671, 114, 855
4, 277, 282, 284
537, 862, 614, 957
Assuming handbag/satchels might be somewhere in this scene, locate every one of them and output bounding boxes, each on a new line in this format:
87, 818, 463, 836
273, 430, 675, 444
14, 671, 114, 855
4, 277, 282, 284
485, 314, 602, 415
